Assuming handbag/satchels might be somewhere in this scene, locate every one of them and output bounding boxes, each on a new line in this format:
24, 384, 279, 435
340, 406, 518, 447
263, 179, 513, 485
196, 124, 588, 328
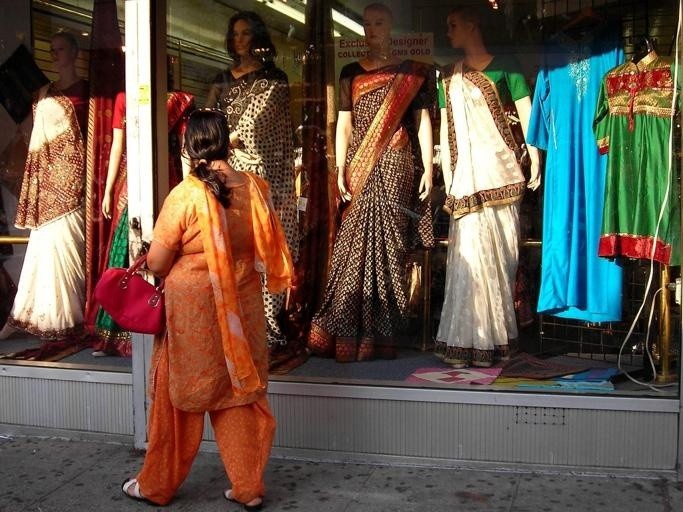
94, 250, 166, 335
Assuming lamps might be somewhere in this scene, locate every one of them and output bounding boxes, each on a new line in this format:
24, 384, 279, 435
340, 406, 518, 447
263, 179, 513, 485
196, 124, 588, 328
331, 7, 366, 40
262, 0, 306, 26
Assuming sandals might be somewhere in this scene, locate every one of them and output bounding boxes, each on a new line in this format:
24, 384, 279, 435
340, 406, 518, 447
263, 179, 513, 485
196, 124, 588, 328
224, 485, 262, 512
123, 478, 158, 505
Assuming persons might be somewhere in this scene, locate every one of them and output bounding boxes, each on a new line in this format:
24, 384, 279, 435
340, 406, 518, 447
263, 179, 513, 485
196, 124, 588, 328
93, 90, 197, 357
439, 10, 543, 368
206, 11, 298, 354
7, 25, 85, 342
307, 3, 433, 361
124, 113, 276, 506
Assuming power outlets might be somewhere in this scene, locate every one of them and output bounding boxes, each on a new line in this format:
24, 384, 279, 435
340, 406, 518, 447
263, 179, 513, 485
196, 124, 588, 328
674, 278, 683, 307
